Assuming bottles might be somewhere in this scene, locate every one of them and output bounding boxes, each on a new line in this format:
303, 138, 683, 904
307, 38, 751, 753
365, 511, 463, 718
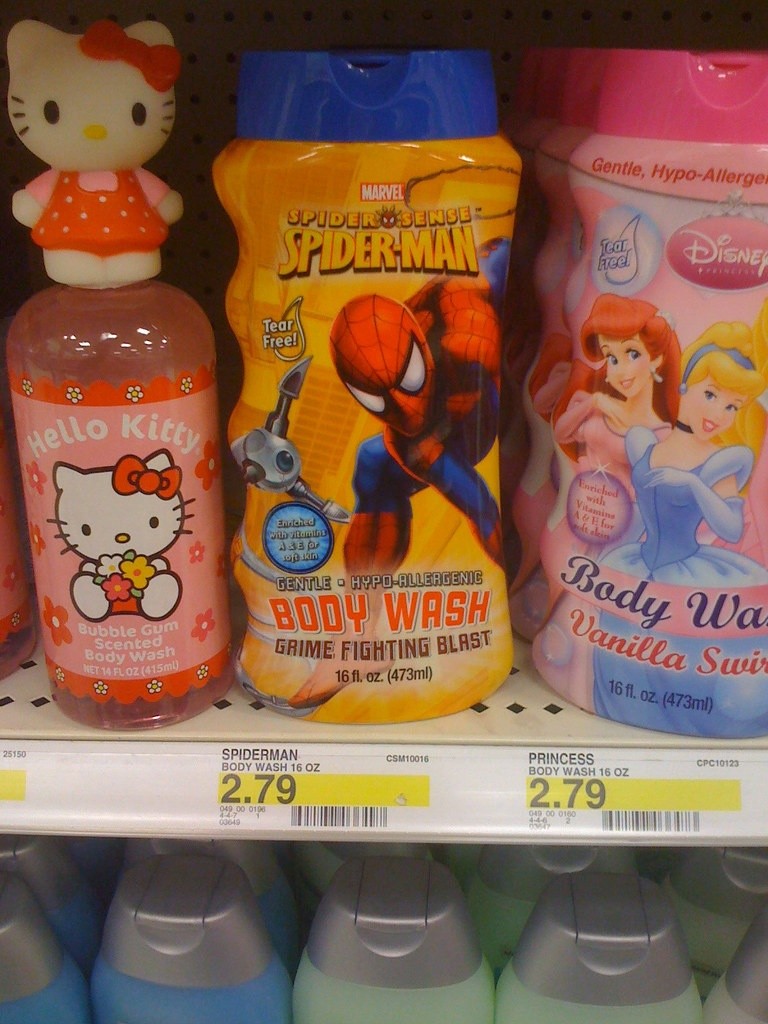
6, 20, 234, 729
212, 45, 520, 722
0, 410, 39, 680
508, 45, 768, 740
